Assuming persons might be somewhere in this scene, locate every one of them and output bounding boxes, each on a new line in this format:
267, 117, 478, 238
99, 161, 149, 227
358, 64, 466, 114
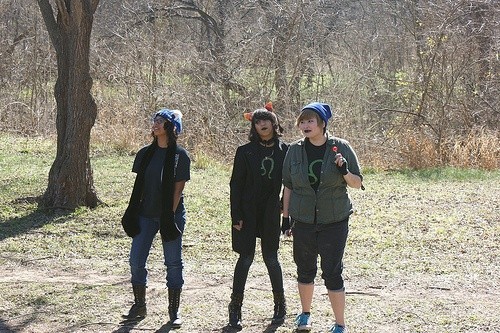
121, 109, 191, 328
282, 103, 365, 333
228, 102, 289, 331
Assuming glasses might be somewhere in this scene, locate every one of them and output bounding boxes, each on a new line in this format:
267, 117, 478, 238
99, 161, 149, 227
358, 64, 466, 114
154, 118, 166, 125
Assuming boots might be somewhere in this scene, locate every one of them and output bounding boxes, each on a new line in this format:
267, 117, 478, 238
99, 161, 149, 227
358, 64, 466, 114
167, 288, 182, 327
128, 286, 147, 318
228, 292, 244, 330
271, 289, 287, 323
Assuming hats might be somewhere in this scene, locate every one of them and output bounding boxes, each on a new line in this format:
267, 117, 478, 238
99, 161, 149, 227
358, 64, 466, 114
153, 109, 183, 137
301, 102, 332, 126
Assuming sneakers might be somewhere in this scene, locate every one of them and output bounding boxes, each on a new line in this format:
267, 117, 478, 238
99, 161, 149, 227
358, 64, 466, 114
294, 313, 313, 330
331, 323, 345, 333
